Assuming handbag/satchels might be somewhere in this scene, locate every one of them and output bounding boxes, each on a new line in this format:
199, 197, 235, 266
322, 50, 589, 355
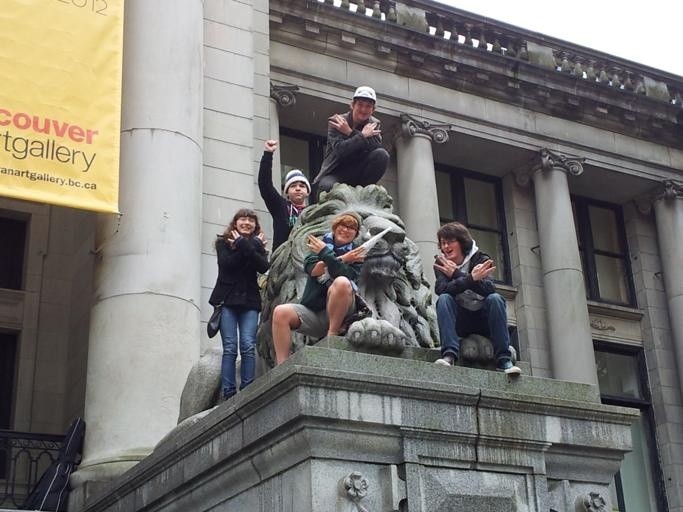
206, 305, 223, 338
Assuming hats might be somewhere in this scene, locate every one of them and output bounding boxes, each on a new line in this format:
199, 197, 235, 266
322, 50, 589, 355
342, 211, 364, 231
352, 85, 376, 103
283, 169, 312, 197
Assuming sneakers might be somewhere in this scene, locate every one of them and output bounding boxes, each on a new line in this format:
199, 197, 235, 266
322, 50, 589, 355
496, 359, 521, 375
434, 354, 455, 366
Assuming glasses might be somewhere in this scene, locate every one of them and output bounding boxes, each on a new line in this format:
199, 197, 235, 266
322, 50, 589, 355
338, 223, 358, 233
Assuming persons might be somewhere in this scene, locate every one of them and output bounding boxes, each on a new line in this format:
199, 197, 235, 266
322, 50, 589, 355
258, 139, 312, 256
272, 213, 368, 364
312, 85, 391, 203
208, 209, 271, 401
433, 221, 522, 375
